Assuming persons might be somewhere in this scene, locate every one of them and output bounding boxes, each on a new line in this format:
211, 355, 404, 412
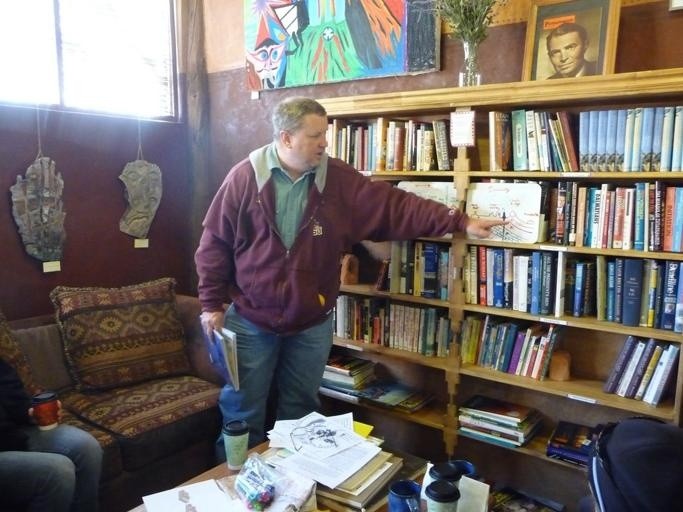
1, 355, 104, 512
192, 96, 510, 461
544, 22, 598, 79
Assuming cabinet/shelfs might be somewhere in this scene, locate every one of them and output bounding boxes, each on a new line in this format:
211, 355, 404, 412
315, 67, 683, 474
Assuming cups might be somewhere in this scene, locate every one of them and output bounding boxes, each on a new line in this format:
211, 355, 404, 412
388, 480, 421, 512
424, 481, 460, 512
30, 393, 59, 430
428, 462, 462, 487
221, 420, 250, 470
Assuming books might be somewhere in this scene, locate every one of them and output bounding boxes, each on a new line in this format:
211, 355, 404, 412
382, 180, 455, 237
490, 180, 682, 253
462, 246, 683, 334
261, 413, 430, 512
512, 105, 682, 172
324, 116, 481, 173
462, 315, 681, 469
204, 326, 239, 392
359, 239, 453, 301
319, 294, 450, 411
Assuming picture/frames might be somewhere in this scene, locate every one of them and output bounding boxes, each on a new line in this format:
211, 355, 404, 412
521, 0, 622, 81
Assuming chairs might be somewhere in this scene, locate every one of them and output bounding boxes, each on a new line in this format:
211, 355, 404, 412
586, 415, 682, 511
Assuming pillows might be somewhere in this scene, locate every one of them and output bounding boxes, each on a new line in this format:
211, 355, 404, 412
49, 275, 193, 392
0, 312, 46, 401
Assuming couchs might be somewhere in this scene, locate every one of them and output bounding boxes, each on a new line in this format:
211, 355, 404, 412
0, 293, 232, 512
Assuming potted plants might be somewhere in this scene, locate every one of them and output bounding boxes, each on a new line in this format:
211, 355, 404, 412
414, 0, 498, 86
